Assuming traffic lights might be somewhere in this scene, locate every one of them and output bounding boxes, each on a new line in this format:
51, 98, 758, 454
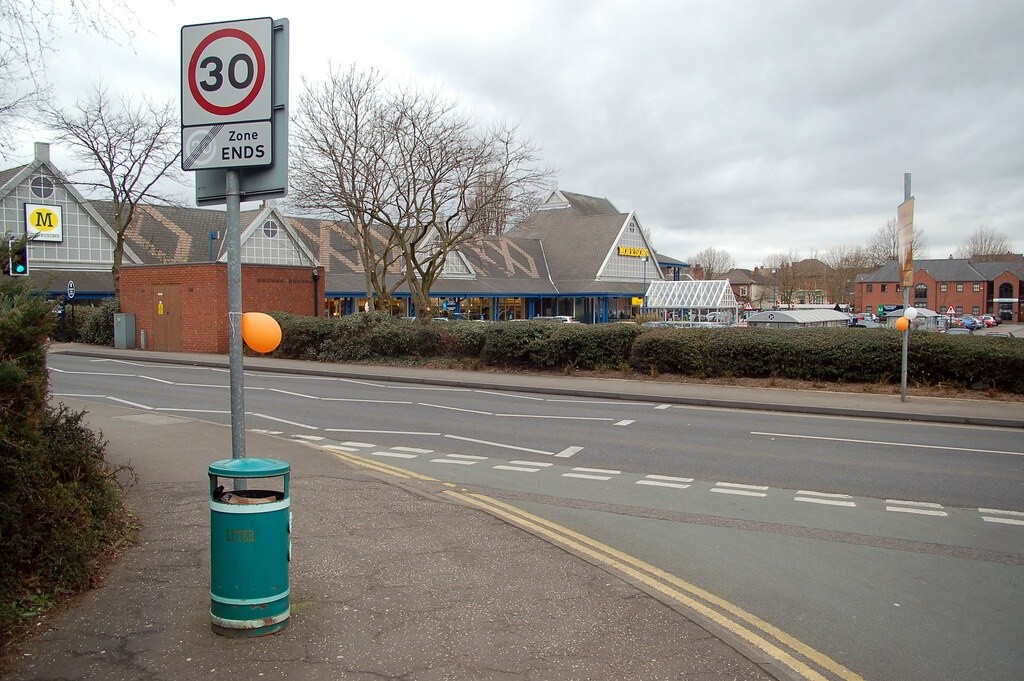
9, 240, 29, 276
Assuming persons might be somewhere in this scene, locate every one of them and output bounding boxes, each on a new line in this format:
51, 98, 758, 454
666, 311, 673, 321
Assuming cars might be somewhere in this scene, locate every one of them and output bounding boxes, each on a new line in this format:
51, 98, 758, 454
985, 331, 1015, 338
843, 312, 887, 323
937, 313, 1002, 330
669, 312, 733, 322
944, 328, 974, 336
401, 316, 580, 326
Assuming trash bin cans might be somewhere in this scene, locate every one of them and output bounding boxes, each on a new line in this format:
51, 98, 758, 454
43, 302, 66, 342
207, 457, 292, 637
853, 318, 858, 323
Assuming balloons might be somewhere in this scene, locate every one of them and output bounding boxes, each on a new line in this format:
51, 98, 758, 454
243, 312, 282, 353
904, 307, 917, 319
895, 317, 909, 331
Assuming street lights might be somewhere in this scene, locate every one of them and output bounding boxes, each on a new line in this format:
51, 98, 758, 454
641, 256, 649, 321
771, 268, 778, 311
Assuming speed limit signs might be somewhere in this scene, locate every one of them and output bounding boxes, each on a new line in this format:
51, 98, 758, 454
187, 29, 266, 117
